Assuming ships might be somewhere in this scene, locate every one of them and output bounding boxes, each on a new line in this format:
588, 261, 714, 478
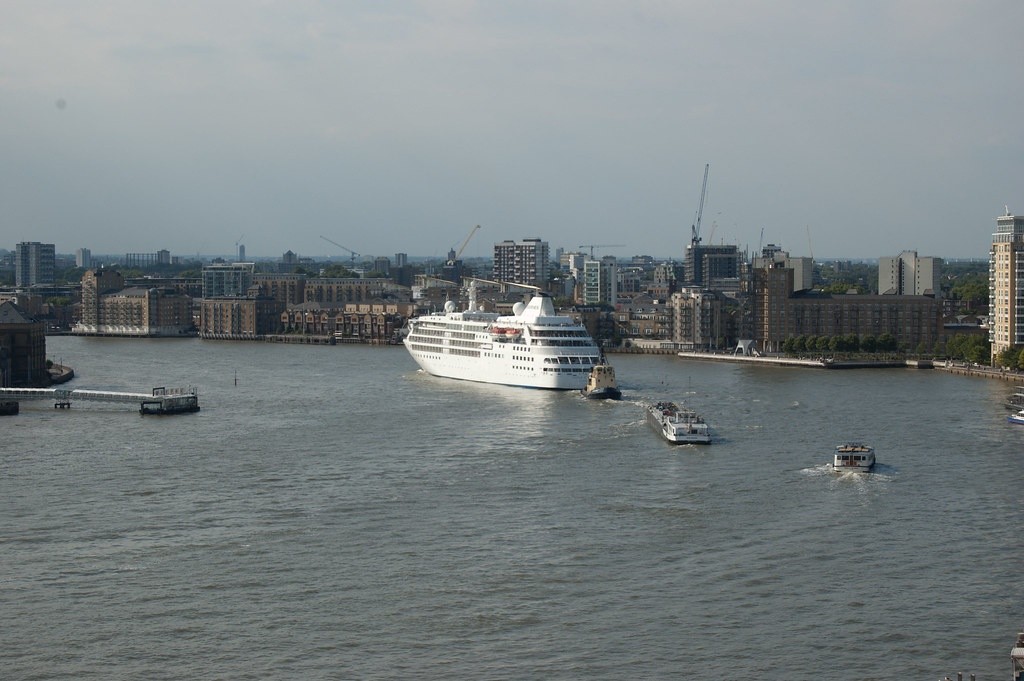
401, 280, 606, 390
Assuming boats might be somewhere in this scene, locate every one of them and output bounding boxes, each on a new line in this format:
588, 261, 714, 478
1008, 410, 1024, 424
580, 346, 621, 401
832, 441, 877, 473
1005, 386, 1024, 410
646, 399, 712, 446
